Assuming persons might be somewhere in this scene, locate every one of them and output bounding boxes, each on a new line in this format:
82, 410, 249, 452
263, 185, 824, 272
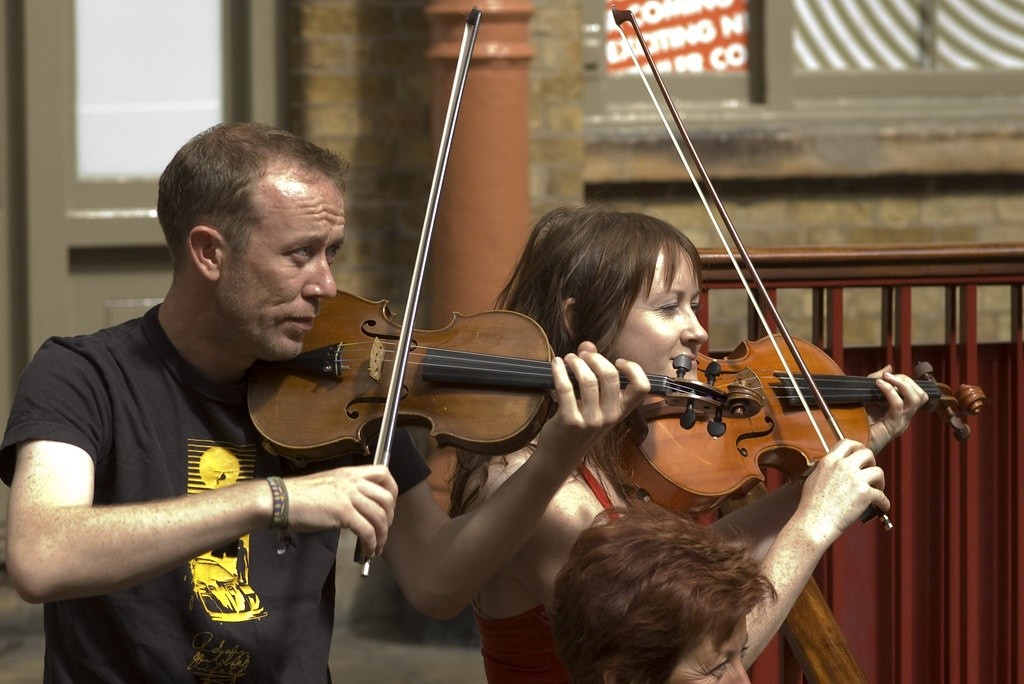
1, 123, 651, 684
547, 499, 777, 684
447, 205, 928, 684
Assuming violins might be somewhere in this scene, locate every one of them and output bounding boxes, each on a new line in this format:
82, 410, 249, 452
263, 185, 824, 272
245, 287, 765, 463
606, 331, 988, 518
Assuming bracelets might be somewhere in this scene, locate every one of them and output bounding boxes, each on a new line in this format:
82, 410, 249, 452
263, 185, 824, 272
266, 477, 288, 530
